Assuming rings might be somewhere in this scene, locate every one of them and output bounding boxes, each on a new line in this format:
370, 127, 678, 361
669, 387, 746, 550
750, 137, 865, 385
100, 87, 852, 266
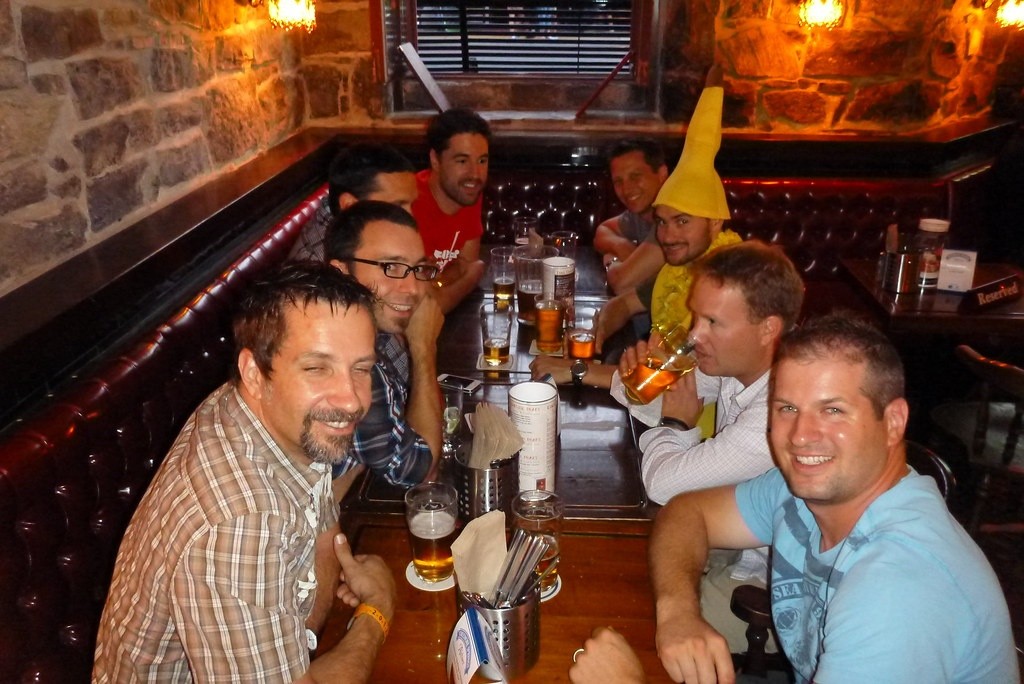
573, 648, 584, 662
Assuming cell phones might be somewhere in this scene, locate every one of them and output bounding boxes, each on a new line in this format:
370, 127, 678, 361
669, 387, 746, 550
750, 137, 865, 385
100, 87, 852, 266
438, 373, 481, 393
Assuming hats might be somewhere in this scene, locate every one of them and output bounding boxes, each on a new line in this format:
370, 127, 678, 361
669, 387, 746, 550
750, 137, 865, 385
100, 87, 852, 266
653, 86, 730, 219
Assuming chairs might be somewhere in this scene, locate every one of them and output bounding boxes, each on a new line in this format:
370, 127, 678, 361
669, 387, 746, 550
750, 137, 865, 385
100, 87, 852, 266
729, 344, 1024, 683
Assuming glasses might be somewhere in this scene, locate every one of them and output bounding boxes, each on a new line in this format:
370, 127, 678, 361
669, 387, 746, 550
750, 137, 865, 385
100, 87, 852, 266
351, 258, 438, 282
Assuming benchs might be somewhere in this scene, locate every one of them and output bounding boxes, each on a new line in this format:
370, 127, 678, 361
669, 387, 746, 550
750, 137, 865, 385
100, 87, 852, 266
0, 181, 337, 684
482, 171, 951, 329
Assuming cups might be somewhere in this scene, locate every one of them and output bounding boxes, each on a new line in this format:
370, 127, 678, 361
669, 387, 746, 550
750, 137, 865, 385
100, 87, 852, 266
534, 293, 566, 353
437, 380, 463, 444
490, 247, 516, 303
622, 325, 707, 405
552, 232, 576, 260
566, 305, 598, 363
513, 217, 537, 247
480, 303, 513, 365
510, 490, 564, 592
513, 245, 559, 325
405, 482, 458, 583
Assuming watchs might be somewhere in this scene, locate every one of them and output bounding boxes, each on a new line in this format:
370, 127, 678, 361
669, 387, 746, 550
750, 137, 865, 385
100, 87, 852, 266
570, 359, 587, 386
305, 629, 318, 656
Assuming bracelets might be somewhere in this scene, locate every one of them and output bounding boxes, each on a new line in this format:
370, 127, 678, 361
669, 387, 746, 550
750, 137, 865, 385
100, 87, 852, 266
660, 416, 689, 430
347, 603, 389, 645
605, 257, 618, 270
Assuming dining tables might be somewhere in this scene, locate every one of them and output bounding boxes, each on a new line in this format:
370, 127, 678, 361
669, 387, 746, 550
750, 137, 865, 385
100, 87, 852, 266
309, 241, 689, 684
834, 254, 1024, 361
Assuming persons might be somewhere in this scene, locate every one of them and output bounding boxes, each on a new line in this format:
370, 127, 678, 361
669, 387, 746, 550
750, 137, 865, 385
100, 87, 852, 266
294, 145, 419, 263
609, 242, 806, 656
412, 106, 493, 316
529, 171, 743, 390
324, 199, 447, 501
593, 136, 667, 296
93, 260, 398, 683
568, 311, 1021, 684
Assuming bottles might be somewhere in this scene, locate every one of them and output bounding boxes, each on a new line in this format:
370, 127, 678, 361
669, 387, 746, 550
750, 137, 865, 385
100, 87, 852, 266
910, 218, 950, 287
875, 252, 887, 281
